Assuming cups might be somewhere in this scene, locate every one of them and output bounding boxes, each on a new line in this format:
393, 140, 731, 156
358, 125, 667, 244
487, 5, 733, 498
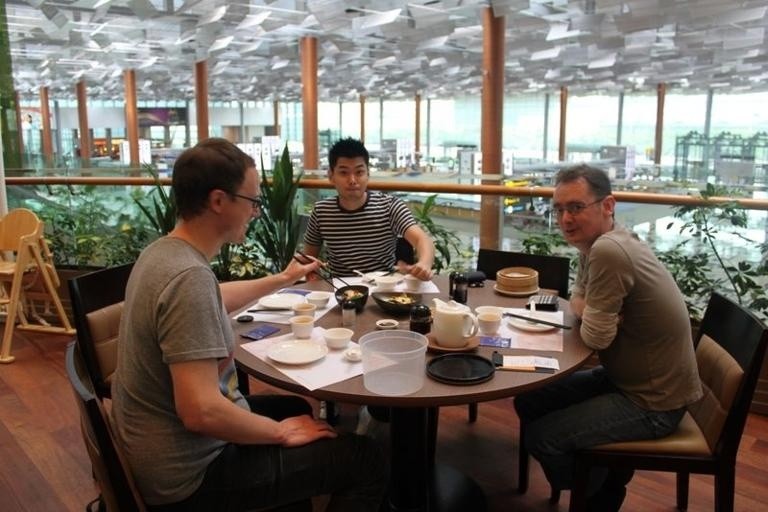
294, 303, 315, 318
403, 274, 425, 292
357, 329, 428, 398
476, 312, 500, 336
290, 316, 314, 338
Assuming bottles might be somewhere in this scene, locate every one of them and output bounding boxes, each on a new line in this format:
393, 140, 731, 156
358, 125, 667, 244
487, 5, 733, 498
448, 270, 466, 304
409, 304, 430, 334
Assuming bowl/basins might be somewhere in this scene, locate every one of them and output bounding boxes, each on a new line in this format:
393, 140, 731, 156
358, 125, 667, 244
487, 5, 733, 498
305, 293, 329, 308
374, 277, 396, 289
321, 327, 356, 349
371, 291, 422, 317
333, 283, 369, 312
473, 303, 504, 319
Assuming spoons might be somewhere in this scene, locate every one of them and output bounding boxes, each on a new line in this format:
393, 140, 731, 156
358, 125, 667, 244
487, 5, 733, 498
525, 300, 539, 324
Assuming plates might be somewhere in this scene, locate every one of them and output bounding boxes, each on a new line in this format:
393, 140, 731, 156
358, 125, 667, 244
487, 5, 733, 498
266, 339, 329, 365
258, 293, 305, 310
424, 329, 480, 353
491, 282, 540, 296
360, 270, 405, 284
375, 318, 398, 330
505, 312, 559, 332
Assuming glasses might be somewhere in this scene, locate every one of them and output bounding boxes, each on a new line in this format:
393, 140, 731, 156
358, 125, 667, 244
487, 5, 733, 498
222, 187, 264, 210
549, 195, 606, 219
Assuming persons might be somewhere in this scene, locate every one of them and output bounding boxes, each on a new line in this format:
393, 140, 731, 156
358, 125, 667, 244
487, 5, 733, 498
513, 163, 703, 512
111, 139, 391, 512
304, 138, 435, 439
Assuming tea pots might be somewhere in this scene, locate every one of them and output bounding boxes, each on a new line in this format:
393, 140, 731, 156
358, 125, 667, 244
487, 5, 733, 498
431, 297, 480, 348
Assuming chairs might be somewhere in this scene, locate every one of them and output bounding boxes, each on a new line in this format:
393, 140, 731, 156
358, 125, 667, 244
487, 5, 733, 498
0, 208, 77, 364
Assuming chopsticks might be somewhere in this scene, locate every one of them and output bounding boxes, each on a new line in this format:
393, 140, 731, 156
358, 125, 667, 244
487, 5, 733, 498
368, 268, 399, 283
245, 308, 291, 313
505, 312, 573, 330
291, 249, 353, 296
